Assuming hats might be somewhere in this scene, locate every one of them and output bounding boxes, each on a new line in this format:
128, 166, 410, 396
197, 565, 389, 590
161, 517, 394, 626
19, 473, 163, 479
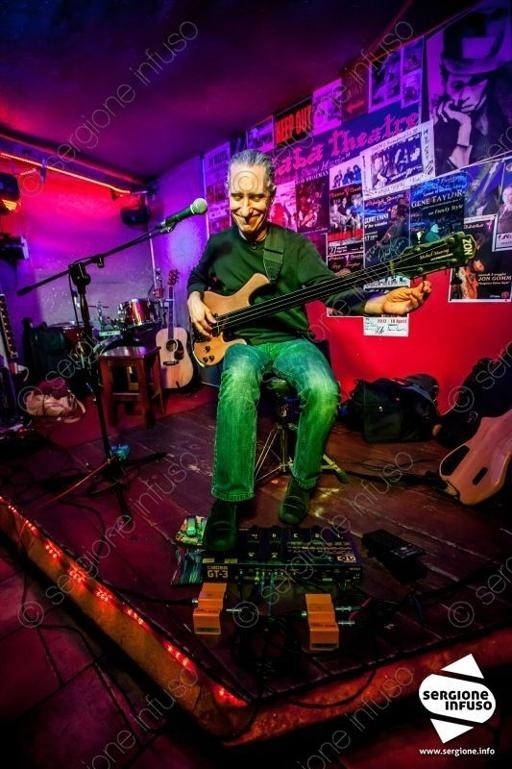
440, 13, 507, 77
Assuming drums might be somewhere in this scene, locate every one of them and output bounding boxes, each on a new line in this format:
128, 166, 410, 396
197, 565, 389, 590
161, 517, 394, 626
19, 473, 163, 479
51, 321, 94, 362
117, 298, 163, 327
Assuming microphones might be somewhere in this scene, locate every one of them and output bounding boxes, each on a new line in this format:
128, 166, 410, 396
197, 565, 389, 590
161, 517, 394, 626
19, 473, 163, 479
156, 197, 208, 228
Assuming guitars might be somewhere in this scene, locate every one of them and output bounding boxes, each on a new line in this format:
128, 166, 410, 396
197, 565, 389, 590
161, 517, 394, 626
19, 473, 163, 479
156, 268, 193, 388
191, 232, 477, 366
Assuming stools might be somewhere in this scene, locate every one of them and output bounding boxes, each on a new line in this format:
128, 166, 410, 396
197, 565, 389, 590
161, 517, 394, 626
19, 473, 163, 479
242, 364, 353, 485
99, 345, 163, 432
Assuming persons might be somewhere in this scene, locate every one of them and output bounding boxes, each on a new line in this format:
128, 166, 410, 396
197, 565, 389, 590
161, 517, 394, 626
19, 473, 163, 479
433, 25, 512, 177
496, 183, 512, 234
332, 164, 362, 188
185, 148, 434, 549
330, 193, 363, 228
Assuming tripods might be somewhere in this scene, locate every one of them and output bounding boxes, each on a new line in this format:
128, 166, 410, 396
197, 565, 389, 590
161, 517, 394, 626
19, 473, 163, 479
64, 261, 165, 527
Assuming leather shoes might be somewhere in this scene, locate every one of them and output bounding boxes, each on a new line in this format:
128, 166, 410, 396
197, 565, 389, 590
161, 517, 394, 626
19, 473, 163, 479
203, 501, 239, 552
278, 479, 312, 525
